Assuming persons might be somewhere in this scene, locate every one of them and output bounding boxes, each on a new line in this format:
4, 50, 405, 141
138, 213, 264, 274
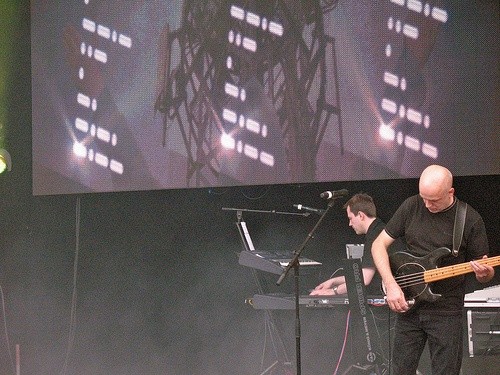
309, 194, 405, 375
370, 164, 494, 375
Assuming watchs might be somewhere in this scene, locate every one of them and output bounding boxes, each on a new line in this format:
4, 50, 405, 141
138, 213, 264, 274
333, 285, 338, 295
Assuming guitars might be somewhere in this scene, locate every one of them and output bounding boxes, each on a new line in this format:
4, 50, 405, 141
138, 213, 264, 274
378, 246, 499, 316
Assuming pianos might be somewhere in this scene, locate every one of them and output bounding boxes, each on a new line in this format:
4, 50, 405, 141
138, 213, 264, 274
252, 286, 319, 312
237, 248, 325, 277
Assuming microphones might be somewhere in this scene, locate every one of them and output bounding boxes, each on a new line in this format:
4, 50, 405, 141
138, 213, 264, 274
319, 189, 349, 200
294, 204, 326, 217
